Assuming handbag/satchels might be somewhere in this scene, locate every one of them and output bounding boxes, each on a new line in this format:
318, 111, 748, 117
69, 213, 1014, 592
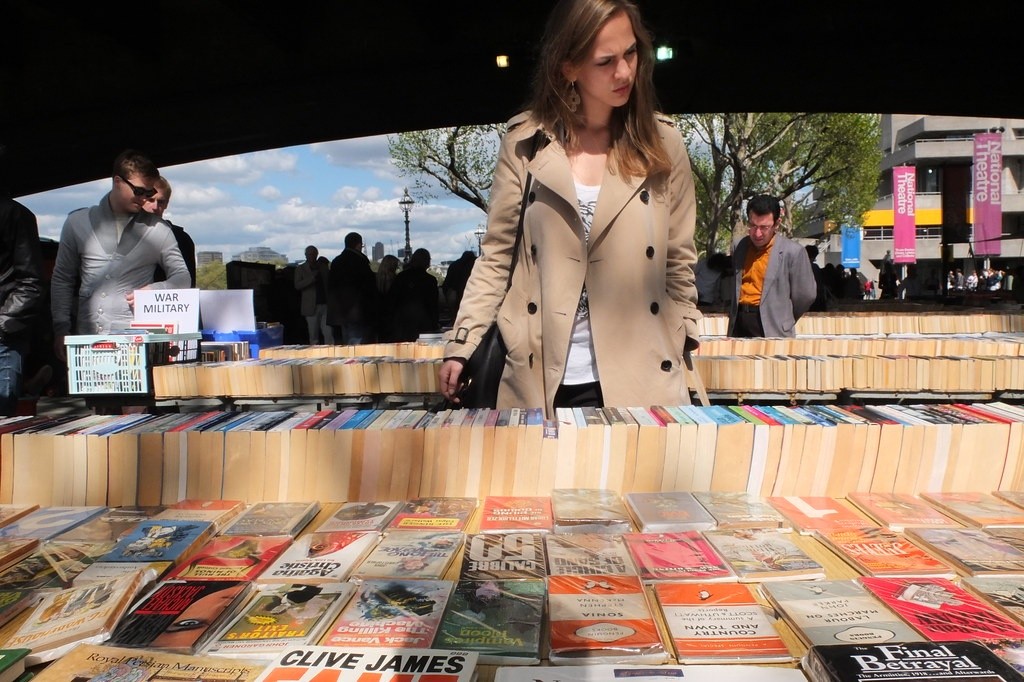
446, 321, 508, 410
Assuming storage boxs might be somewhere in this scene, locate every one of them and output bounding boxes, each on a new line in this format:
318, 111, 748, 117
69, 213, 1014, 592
64, 331, 168, 394
200, 324, 285, 358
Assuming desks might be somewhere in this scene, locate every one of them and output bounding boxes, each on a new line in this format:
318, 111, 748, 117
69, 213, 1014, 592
54, 392, 1024, 408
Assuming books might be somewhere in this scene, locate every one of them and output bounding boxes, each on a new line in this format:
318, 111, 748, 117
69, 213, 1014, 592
0, 401, 1024, 682
686, 336, 1024, 390
152, 341, 447, 397
699, 311, 1024, 336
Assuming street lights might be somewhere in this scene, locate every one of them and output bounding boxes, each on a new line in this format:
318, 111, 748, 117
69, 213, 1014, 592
473, 227, 486, 256
398, 187, 414, 270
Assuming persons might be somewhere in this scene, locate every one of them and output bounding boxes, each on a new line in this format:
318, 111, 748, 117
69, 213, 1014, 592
726, 194, 818, 338
438, 0, 710, 410
0, 147, 196, 417
292, 231, 477, 343
693, 249, 1006, 306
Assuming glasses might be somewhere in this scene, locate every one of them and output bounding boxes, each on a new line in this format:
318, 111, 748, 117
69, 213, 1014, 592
747, 219, 777, 231
121, 176, 158, 198
